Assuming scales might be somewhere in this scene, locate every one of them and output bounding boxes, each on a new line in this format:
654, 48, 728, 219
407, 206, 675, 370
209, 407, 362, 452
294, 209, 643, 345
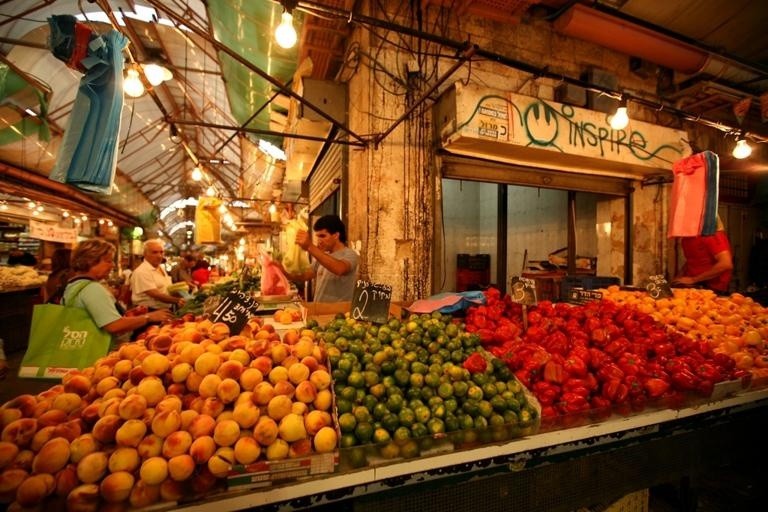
254, 297, 291, 315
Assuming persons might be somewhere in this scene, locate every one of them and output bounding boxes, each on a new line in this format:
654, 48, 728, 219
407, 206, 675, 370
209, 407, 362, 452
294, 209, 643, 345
268, 214, 360, 303
39, 238, 225, 382
7, 247, 38, 267
669, 213, 734, 297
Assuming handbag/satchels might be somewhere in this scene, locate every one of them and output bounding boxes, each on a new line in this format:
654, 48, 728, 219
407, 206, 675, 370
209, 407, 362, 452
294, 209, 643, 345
14, 280, 113, 383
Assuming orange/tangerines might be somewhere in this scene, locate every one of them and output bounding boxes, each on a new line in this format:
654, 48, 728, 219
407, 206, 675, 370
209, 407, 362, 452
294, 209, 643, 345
306, 311, 537, 469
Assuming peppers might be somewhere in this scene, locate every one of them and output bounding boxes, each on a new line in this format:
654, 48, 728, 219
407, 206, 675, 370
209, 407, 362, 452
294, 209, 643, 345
464, 285, 768, 429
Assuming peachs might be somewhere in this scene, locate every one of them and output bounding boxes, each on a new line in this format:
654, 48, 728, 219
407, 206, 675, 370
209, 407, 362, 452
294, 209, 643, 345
0, 314, 338, 512
